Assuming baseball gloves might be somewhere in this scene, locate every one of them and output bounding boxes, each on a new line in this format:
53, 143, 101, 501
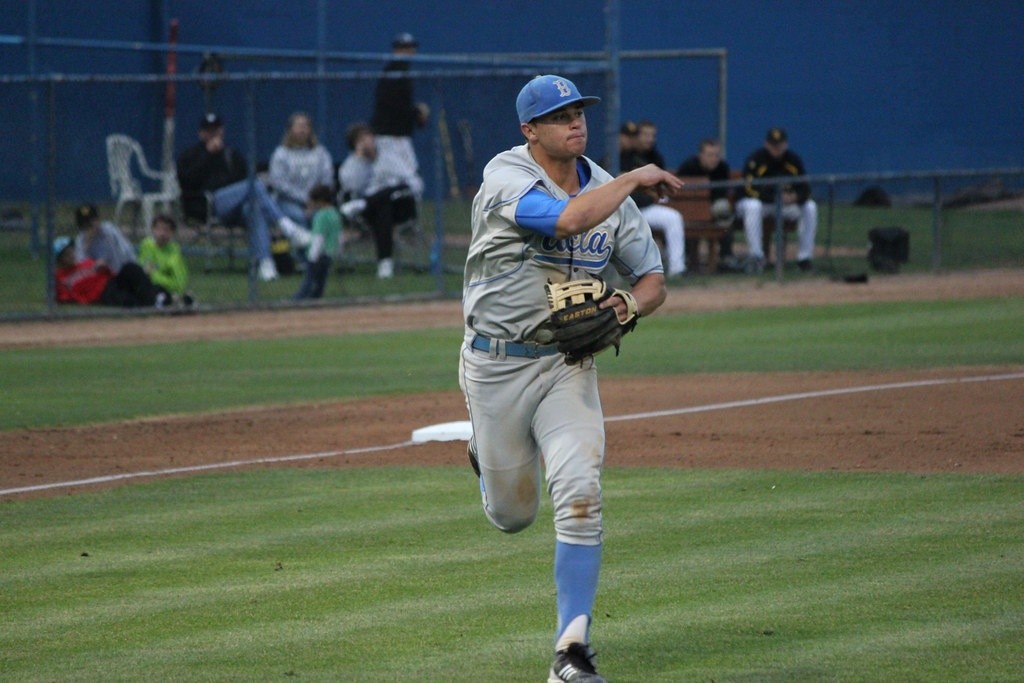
544, 279, 637, 365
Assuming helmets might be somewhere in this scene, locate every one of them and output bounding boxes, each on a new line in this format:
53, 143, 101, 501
51, 235, 74, 258
391, 32, 419, 48
711, 198, 736, 229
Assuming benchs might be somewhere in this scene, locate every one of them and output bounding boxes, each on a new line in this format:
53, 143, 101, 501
667, 171, 797, 276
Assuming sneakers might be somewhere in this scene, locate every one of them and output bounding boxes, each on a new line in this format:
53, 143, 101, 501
546, 642, 604, 683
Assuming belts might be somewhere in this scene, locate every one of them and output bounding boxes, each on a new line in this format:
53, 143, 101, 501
472, 335, 561, 358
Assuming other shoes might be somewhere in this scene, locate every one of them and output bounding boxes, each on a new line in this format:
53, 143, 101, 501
374, 257, 395, 278
256, 259, 280, 282
183, 294, 193, 305
798, 260, 812, 271
154, 292, 170, 308
339, 201, 357, 223
714, 251, 737, 270
288, 226, 312, 249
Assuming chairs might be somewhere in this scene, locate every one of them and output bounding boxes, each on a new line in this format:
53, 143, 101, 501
180, 190, 425, 276
106, 135, 179, 236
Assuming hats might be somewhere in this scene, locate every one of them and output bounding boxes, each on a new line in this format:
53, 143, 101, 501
200, 113, 223, 131
516, 75, 602, 126
766, 127, 787, 145
621, 121, 641, 136
74, 200, 99, 231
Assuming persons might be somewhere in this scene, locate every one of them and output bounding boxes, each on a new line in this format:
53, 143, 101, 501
599, 120, 739, 278
459, 74, 684, 683
52, 33, 428, 310
733, 127, 817, 275
